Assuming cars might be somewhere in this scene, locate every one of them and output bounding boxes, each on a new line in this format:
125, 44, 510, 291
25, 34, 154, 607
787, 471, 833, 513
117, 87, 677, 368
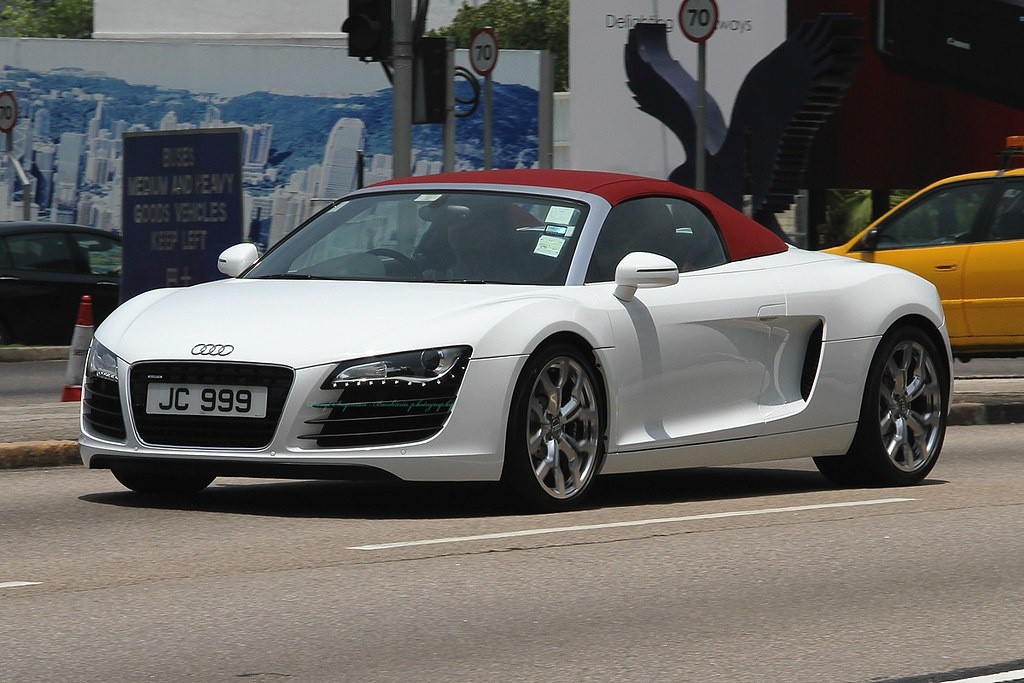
0, 220, 123, 346
805, 136, 1024, 363
77, 171, 954, 509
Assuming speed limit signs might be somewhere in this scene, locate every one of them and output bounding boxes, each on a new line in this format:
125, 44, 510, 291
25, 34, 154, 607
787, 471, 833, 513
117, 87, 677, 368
678, 0, 718, 42
470, 31, 497, 77
0, 92, 17, 132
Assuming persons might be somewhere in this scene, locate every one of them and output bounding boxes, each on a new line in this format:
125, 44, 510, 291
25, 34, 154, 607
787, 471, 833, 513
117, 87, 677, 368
416, 205, 513, 284
527, 210, 662, 284
